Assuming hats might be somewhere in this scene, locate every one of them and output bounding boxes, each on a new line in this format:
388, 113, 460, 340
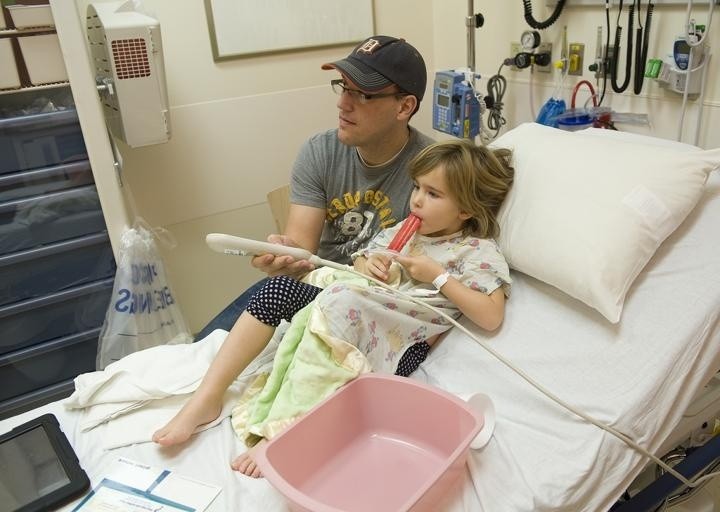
321, 34, 427, 103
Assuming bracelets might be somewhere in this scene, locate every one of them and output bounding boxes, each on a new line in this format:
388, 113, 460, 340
431, 270, 452, 290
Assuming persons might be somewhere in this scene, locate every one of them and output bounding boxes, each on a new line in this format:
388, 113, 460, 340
192, 34, 442, 343
152, 138, 515, 478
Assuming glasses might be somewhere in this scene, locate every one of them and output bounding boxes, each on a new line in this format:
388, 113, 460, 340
328, 77, 408, 101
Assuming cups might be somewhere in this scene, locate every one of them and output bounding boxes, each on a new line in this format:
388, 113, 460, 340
558, 113, 595, 131
461, 391, 496, 450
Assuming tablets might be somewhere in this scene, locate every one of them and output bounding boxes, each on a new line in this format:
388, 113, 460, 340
1, 413, 90, 512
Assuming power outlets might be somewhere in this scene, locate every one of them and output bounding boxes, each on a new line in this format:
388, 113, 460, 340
535, 37, 553, 73
558, 43, 585, 76
508, 40, 528, 71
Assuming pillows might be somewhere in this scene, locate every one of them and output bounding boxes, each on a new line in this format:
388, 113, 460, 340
477, 119, 720, 330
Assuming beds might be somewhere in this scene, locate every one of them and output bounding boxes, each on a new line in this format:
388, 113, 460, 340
0, 118, 720, 512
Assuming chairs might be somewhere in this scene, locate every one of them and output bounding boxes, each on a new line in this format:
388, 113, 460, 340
263, 180, 292, 239
